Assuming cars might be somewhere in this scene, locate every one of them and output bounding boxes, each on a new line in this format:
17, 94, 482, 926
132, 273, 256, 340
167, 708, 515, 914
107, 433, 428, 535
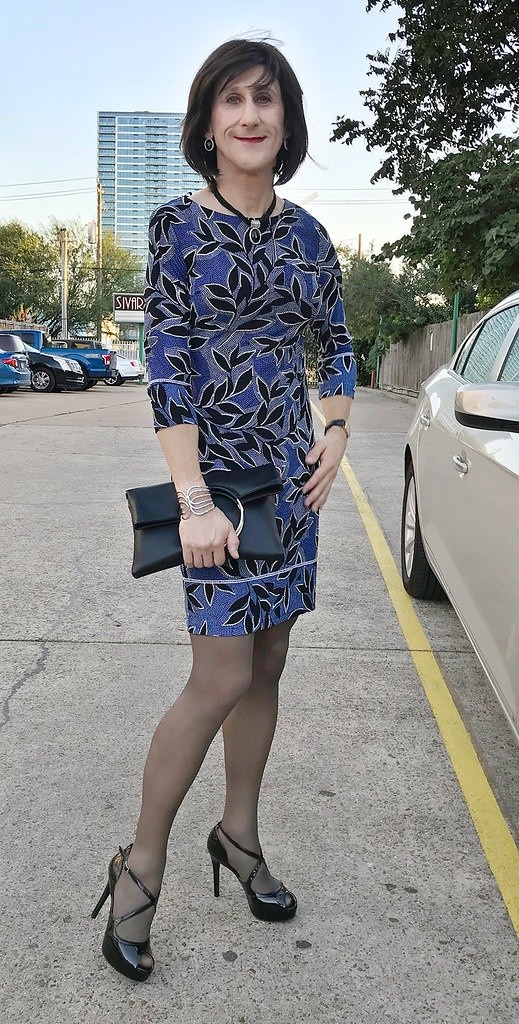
399, 288, 519, 745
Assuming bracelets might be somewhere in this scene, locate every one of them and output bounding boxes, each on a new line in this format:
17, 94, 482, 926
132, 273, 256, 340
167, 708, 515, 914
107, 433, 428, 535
177, 486, 215, 520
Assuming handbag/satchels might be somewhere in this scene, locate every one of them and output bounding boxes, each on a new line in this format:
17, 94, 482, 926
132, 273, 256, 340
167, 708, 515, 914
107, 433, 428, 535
126, 463, 286, 579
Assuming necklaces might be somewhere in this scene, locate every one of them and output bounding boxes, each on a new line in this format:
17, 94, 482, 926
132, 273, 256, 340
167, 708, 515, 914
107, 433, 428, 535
207, 178, 278, 244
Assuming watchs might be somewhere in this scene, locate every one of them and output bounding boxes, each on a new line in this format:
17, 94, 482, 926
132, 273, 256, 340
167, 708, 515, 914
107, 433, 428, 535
325, 419, 352, 438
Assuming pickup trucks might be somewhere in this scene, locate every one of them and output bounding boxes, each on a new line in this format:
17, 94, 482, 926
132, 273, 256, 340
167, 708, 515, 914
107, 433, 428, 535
0, 329, 144, 392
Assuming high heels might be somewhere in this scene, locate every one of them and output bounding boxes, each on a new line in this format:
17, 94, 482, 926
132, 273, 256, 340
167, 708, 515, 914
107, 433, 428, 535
207, 821, 297, 921
91, 843, 160, 982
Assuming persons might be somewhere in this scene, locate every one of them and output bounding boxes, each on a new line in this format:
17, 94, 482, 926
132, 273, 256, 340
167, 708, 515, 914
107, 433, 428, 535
90, 39, 358, 982
